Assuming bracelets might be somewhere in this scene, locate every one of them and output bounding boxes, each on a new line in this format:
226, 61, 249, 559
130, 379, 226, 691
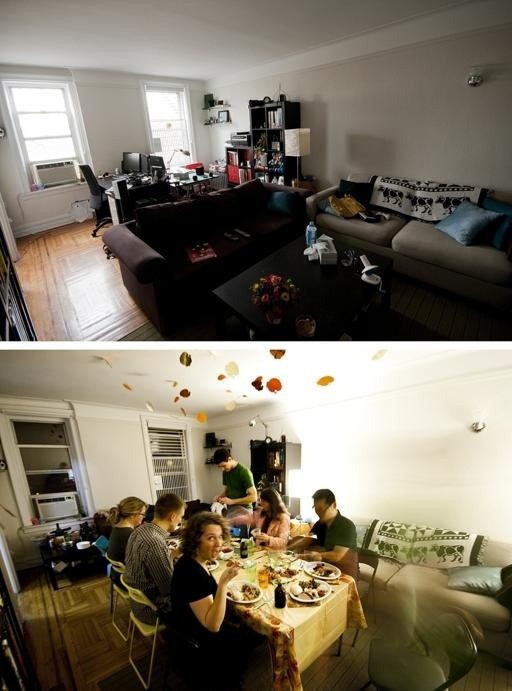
218, 546, 235, 561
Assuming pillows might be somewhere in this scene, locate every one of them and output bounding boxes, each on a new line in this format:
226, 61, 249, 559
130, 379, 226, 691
477, 194, 512, 250
447, 566, 503, 594
269, 190, 295, 215
438, 201, 504, 245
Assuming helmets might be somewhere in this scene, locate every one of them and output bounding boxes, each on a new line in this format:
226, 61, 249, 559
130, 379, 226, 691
201, 557, 219, 571
302, 560, 342, 580
76, 539, 90, 551
286, 579, 333, 603
223, 579, 264, 605
282, 550, 298, 563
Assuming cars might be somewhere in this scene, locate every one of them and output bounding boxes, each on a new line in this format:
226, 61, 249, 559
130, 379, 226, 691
250, 528, 262, 538
258, 571, 269, 589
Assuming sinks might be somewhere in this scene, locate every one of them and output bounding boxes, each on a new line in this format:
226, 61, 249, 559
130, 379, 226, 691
102, 178, 312, 337
350, 521, 512, 667
306, 174, 512, 312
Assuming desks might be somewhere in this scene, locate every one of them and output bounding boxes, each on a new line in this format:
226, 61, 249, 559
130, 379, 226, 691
169, 172, 219, 200
97, 176, 169, 206
39, 537, 104, 589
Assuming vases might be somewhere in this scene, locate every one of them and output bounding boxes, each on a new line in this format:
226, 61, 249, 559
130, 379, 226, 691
249, 273, 301, 308
264, 307, 286, 325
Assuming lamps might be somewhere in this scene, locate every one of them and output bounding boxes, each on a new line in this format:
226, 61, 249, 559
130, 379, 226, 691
467, 65, 483, 87
284, 128, 311, 187
287, 468, 311, 522
79, 165, 112, 236
360, 612, 477, 691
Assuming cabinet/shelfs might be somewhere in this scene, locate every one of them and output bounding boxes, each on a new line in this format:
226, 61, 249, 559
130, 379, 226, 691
249, 101, 302, 186
273, 581, 287, 608
250, 439, 301, 517
226, 147, 251, 186
305, 220, 318, 248
55, 523, 64, 537
241, 538, 248, 558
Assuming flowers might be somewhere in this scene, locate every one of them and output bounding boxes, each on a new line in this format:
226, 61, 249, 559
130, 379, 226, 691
249, 273, 301, 308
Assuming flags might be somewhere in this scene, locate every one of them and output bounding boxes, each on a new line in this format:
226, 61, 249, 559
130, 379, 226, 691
264, 307, 286, 325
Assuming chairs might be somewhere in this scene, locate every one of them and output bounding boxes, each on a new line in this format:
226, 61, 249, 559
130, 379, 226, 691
360, 612, 477, 691
104, 508, 378, 691
79, 165, 112, 236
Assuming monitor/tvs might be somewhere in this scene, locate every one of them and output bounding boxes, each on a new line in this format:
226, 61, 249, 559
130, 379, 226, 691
228, 150, 251, 184
268, 108, 282, 128
187, 241, 217, 262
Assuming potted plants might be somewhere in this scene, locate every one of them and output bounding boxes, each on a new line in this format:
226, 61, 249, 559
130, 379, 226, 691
218, 110, 230, 122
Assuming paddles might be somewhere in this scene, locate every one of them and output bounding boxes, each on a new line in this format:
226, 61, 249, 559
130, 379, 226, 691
111, 274, 512, 341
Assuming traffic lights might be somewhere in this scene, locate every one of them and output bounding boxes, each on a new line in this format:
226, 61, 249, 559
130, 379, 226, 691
477, 194, 512, 250
447, 566, 503, 594
269, 190, 295, 215
438, 201, 504, 245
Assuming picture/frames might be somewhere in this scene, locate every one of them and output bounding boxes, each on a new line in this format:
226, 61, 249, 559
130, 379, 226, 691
39, 537, 104, 589
218, 110, 230, 122
97, 176, 169, 206
169, 172, 219, 200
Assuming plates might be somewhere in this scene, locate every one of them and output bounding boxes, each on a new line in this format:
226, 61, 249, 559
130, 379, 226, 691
250, 439, 301, 517
249, 101, 302, 186
226, 147, 251, 186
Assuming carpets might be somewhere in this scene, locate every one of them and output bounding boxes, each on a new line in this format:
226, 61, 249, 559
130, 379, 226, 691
111, 274, 512, 341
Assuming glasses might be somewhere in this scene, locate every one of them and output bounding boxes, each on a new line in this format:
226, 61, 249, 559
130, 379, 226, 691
284, 128, 311, 187
287, 468, 311, 522
467, 65, 483, 87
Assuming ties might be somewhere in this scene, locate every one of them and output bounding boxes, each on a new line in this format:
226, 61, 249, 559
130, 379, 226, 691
121, 152, 167, 178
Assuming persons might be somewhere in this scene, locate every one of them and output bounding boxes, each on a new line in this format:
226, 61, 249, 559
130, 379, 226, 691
224, 488, 289, 552
287, 489, 359, 585
56, 464, 68, 484
211, 449, 257, 538
124, 492, 186, 625
107, 496, 149, 591
165, 510, 268, 673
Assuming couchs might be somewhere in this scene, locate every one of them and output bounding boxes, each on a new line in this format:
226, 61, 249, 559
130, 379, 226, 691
350, 521, 512, 667
102, 178, 312, 337
306, 174, 512, 312
